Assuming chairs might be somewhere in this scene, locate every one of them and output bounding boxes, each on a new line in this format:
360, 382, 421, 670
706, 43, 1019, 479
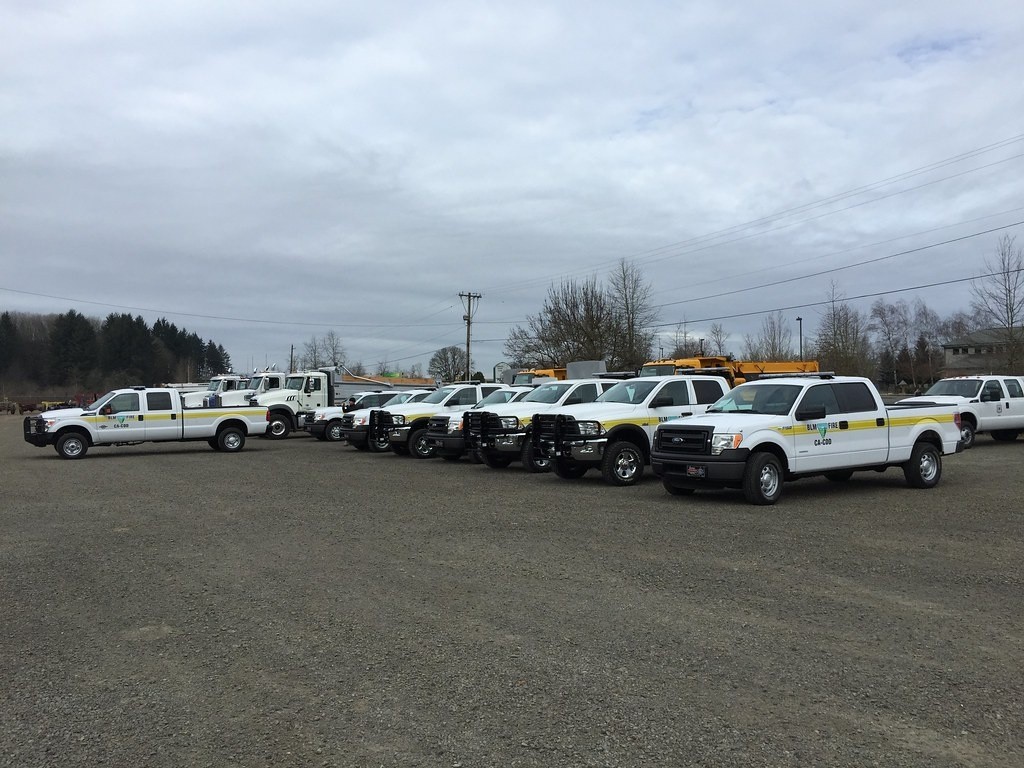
1007, 384, 1017, 393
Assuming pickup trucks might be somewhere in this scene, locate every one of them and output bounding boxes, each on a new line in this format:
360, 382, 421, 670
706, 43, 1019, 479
649, 372, 965, 506
893, 376, 1024, 449
23, 386, 271, 460
304, 383, 510, 465
461, 371, 753, 485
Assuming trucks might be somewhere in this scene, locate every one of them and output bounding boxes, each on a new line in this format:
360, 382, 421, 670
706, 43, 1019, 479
639, 357, 823, 391
512, 369, 567, 384
180, 363, 452, 440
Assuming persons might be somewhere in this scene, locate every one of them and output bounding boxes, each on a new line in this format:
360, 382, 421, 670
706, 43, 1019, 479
343, 398, 358, 445
